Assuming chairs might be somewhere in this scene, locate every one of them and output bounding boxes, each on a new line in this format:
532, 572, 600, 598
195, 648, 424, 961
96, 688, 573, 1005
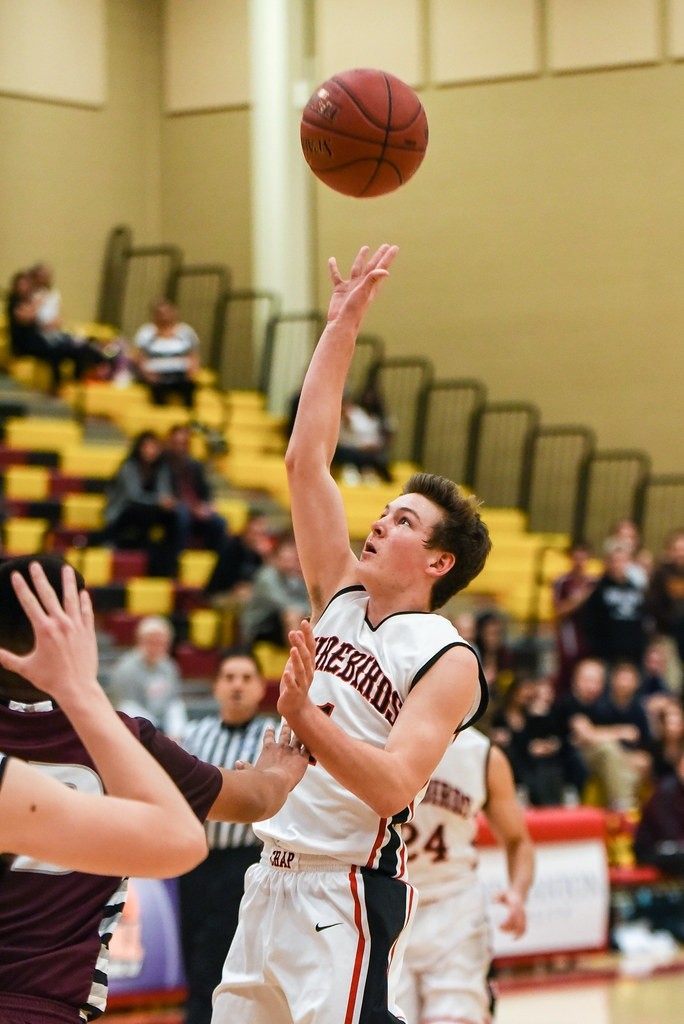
1, 320, 606, 712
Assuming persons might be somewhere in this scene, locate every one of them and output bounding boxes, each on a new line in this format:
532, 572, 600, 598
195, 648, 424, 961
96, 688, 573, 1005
209, 243, 488, 1024
0, 550, 311, 1024
392, 723, 536, 1023
96, 424, 226, 580
7, 264, 107, 391
210, 507, 275, 598
131, 299, 201, 409
236, 537, 314, 648
0, 561, 208, 880
473, 516, 684, 881
169, 644, 283, 1023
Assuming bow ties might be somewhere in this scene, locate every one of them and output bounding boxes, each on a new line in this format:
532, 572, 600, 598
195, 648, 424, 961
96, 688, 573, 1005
222, 719, 252, 733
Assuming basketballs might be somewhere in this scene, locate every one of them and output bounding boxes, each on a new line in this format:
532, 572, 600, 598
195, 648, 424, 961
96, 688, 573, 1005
300, 67, 430, 200
